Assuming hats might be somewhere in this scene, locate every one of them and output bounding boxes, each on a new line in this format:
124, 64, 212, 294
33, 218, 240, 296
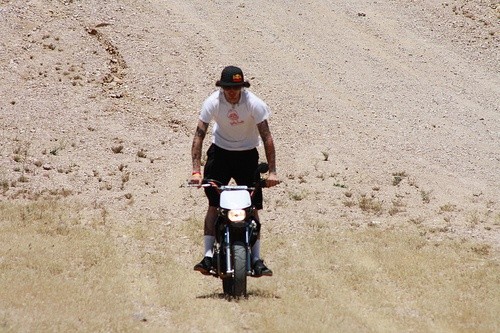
215, 66, 251, 87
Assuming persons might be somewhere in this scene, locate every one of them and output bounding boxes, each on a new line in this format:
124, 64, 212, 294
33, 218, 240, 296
188, 66, 281, 276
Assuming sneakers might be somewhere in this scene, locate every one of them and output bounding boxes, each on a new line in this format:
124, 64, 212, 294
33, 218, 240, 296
194, 256, 214, 275
251, 259, 272, 276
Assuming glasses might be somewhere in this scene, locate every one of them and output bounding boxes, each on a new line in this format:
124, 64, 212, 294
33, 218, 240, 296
223, 86, 241, 90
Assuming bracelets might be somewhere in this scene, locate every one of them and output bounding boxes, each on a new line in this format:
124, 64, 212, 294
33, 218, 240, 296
191, 170, 202, 175
193, 174, 203, 177
269, 169, 277, 173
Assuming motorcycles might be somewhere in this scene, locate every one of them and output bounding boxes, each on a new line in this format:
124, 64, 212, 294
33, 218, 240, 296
179, 162, 283, 302
